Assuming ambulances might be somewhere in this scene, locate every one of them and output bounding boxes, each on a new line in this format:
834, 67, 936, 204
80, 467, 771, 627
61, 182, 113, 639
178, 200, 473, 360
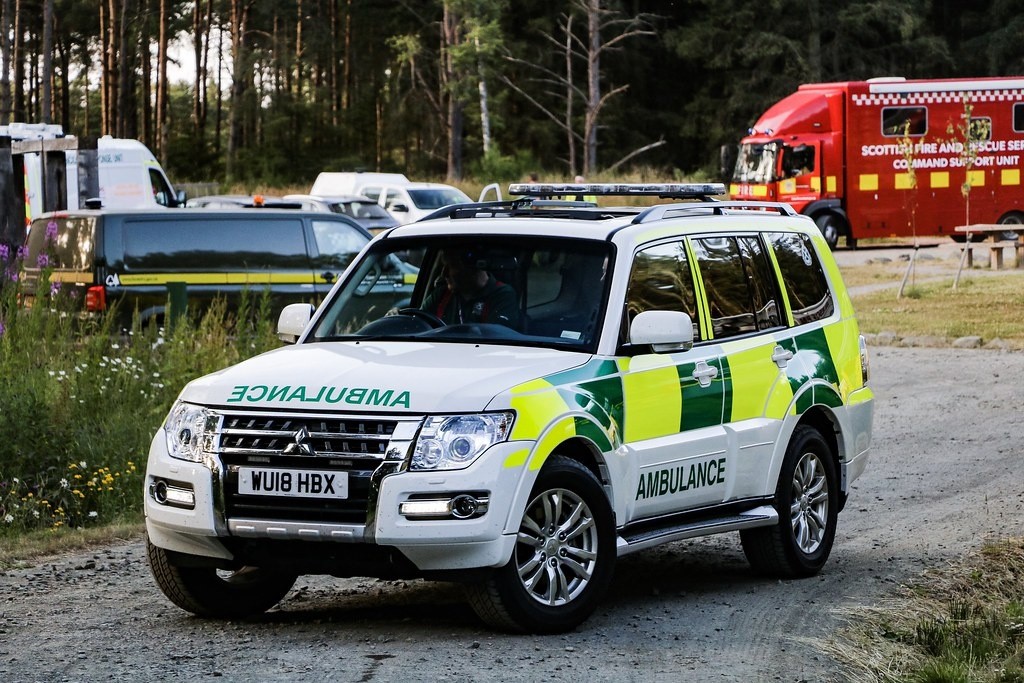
143, 182, 876, 636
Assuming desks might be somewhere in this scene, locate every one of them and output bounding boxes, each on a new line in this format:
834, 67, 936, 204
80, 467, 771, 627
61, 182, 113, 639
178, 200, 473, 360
954, 224, 1024, 268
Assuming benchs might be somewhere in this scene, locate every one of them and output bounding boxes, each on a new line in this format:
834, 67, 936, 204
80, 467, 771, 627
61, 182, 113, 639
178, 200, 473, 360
960, 241, 1024, 270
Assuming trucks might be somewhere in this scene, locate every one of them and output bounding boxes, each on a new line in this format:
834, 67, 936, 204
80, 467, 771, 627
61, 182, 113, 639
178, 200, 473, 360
729, 76, 1024, 256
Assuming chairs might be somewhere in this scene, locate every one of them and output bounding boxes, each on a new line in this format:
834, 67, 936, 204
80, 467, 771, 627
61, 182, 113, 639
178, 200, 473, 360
489, 254, 534, 336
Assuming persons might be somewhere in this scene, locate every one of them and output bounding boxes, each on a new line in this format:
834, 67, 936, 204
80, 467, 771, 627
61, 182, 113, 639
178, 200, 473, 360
565, 175, 598, 204
416, 243, 523, 331
519, 174, 551, 201
789, 155, 810, 180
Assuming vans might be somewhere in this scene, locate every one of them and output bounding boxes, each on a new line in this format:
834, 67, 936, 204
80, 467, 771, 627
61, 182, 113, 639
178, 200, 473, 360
1, 123, 516, 360
